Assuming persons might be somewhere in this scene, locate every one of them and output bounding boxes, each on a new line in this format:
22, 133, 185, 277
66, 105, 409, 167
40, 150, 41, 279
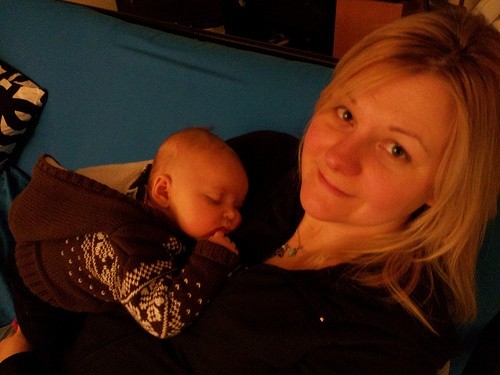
0, 7, 500, 374
10, 128, 250, 375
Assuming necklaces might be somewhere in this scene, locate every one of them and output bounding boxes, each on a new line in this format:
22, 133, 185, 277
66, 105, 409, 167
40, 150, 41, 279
275, 227, 304, 257
